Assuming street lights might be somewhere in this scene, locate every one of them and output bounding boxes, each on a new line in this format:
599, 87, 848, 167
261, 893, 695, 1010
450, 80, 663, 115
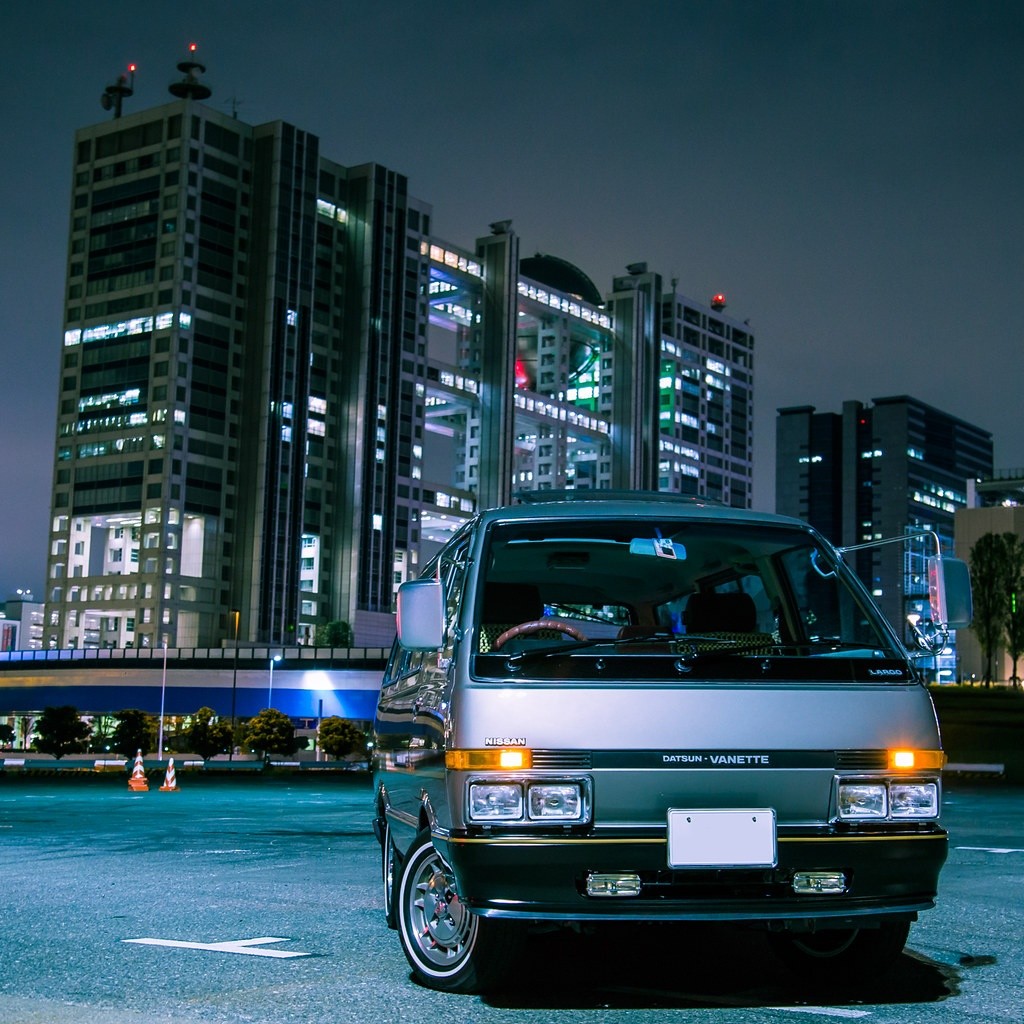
158, 643, 168, 761
268, 655, 281, 709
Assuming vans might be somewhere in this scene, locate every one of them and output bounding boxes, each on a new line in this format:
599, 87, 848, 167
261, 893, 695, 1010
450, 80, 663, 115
368, 488, 951, 993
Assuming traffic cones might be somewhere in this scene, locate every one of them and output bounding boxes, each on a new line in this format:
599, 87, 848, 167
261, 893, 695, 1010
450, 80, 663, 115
160, 757, 181, 792
127, 748, 150, 792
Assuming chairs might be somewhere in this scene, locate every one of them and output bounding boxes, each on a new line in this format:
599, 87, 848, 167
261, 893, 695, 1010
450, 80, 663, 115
683, 592, 757, 633
618, 626, 662, 640
481, 580, 544, 623
